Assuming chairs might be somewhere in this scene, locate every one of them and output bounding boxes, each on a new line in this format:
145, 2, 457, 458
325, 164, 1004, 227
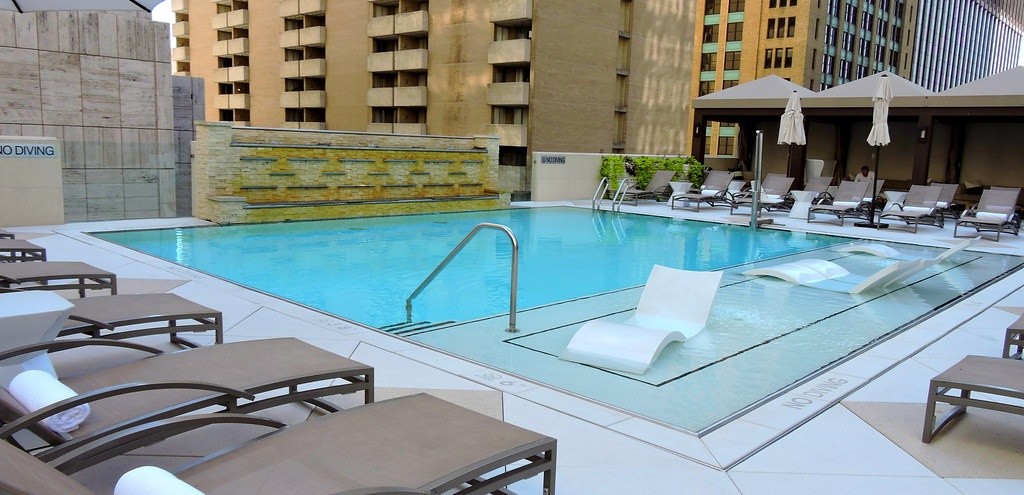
878, 184, 944, 235
954, 189, 1020, 241
731, 177, 796, 218
852, 180, 887, 218
921, 315, 1024, 446
831, 236, 983, 266
732, 173, 787, 208
807, 181, 871, 227
787, 176, 834, 211
969, 186, 1022, 230
670, 173, 738, 213
564, 264, 724, 379
923, 182, 961, 226
788, 251, 946, 285
743, 257, 923, 297
689, 170, 731, 194
0, 230, 558, 495
616, 170, 677, 206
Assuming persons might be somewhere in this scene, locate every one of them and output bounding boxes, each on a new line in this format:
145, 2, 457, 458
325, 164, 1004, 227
854, 166, 874, 183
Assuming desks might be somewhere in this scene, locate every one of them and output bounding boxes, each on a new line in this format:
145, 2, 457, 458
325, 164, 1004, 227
883, 190, 908, 220
668, 182, 694, 207
726, 181, 746, 203
789, 190, 819, 220
0, 290, 76, 392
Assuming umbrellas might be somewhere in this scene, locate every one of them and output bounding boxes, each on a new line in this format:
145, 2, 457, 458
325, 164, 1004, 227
867, 74, 893, 224
777, 90, 807, 178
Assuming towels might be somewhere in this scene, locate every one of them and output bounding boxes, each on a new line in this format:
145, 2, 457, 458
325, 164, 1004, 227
114, 465, 205, 495
8, 370, 91, 433
